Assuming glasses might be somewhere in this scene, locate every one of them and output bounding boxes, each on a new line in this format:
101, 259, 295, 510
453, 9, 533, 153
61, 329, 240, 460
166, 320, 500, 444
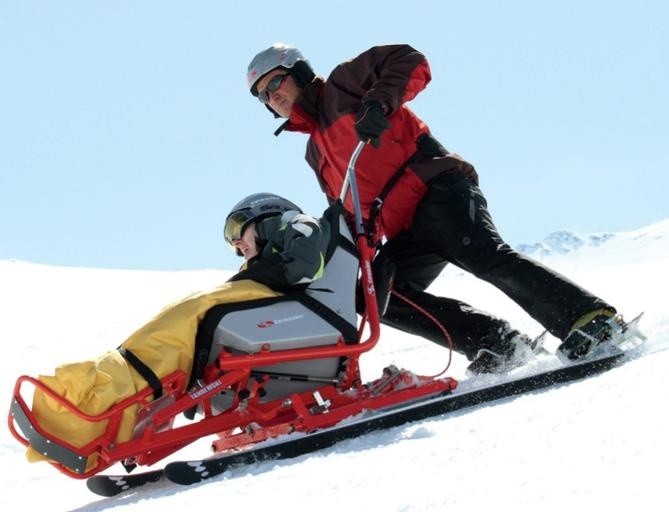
257, 72, 290, 104
224, 201, 298, 245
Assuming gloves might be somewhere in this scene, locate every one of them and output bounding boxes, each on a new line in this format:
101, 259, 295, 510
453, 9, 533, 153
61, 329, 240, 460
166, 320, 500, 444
353, 101, 392, 149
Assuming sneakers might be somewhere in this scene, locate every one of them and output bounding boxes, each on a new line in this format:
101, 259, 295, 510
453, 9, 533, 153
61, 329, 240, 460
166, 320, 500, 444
468, 329, 529, 373
562, 308, 617, 352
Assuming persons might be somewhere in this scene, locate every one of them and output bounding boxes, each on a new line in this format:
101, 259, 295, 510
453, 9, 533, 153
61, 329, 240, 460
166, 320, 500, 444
26, 193, 331, 474
247, 44, 627, 377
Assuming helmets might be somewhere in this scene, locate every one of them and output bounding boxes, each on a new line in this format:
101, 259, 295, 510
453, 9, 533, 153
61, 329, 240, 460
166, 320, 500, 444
246, 43, 316, 94
227, 193, 301, 255
